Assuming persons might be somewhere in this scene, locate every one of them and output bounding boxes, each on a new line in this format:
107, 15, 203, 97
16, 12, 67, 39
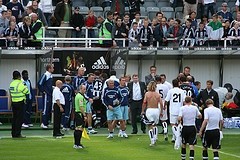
141, 81, 163, 147
9, 71, 30, 138
0, 0, 240, 48
199, 99, 223, 160
73, 85, 87, 149
21, 64, 237, 150
178, 97, 202, 160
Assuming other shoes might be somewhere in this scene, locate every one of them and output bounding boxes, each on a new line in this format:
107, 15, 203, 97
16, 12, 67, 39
41, 123, 48, 128
118, 132, 121, 137
54, 133, 61, 136
165, 137, 168, 141
131, 132, 137, 134
120, 133, 128, 138
22, 123, 29, 127
28, 124, 33, 126
73, 145, 82, 148
87, 129, 97, 134
171, 140, 175, 143
60, 132, 64, 136
97, 126, 100, 129
159, 131, 164, 134
13, 135, 26, 138
143, 132, 146, 133
107, 133, 113, 138
70, 125, 75, 130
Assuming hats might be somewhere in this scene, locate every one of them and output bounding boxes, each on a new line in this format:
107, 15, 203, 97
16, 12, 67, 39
75, 6, 80, 11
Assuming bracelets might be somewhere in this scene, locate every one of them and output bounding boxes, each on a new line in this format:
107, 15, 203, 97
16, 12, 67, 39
84, 116, 87, 117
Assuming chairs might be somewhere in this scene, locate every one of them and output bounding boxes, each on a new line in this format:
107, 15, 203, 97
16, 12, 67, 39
0, 87, 43, 127
68, 0, 240, 37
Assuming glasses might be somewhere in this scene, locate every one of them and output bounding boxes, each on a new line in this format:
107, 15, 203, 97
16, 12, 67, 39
222, 6, 227, 7
